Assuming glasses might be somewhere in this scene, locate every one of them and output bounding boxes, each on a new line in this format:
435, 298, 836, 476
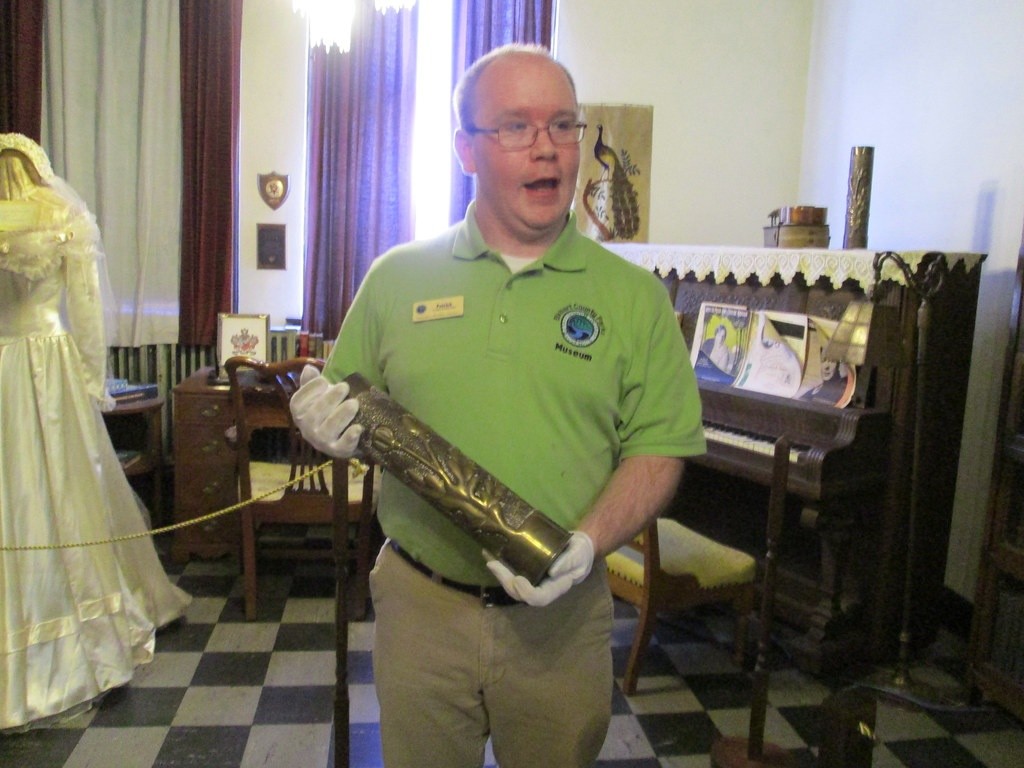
468, 119, 588, 147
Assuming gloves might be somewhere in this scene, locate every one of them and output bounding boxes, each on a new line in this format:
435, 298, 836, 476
291, 363, 362, 460
482, 529, 594, 608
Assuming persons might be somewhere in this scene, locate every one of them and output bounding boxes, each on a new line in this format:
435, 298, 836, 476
702, 325, 729, 373
800, 347, 848, 406
0, 134, 193, 737
288, 44, 707, 768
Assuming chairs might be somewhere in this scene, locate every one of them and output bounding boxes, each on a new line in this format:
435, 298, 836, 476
604, 516, 759, 698
221, 357, 384, 622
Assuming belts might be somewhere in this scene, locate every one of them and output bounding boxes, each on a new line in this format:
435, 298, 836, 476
389, 541, 529, 606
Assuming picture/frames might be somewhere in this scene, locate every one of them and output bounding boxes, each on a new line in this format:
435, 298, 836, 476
216, 311, 271, 385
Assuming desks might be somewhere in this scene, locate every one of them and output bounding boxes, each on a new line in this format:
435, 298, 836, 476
97, 395, 165, 541
170, 362, 328, 561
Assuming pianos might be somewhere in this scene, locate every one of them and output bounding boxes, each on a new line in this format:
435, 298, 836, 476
600, 242, 990, 677
